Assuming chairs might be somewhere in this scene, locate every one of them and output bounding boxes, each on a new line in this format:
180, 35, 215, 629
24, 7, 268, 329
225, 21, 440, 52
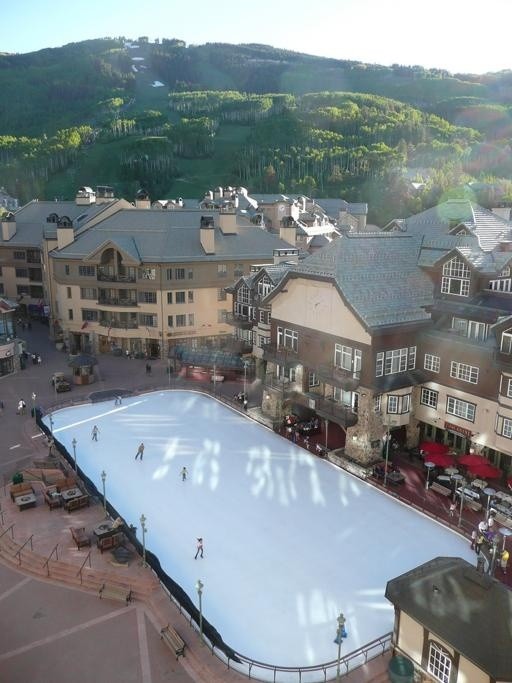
8, 479, 127, 555
299, 422, 512, 530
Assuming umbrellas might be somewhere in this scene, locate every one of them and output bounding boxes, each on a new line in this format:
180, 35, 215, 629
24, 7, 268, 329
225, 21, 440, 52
418, 441, 512, 489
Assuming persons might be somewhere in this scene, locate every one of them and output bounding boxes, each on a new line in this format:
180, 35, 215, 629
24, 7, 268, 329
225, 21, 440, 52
336, 613, 346, 637
115, 395, 122, 405
180, 467, 189, 481
283, 416, 320, 449
233, 392, 248, 411
125, 348, 151, 376
30, 352, 42, 365
91, 425, 101, 441
19, 398, 25, 416
134, 443, 144, 460
194, 538, 204, 560
449, 502, 510, 575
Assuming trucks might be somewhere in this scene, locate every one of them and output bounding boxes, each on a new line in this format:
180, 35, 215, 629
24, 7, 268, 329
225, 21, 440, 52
49, 370, 71, 393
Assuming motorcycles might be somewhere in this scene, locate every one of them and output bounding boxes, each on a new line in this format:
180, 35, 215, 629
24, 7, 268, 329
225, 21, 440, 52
31, 353, 42, 365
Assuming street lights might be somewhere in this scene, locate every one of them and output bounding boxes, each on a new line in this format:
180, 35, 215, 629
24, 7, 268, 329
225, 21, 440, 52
100, 468, 108, 518
335, 612, 347, 678
167, 358, 173, 385
70, 435, 78, 473
382, 426, 392, 488
141, 512, 150, 567
213, 363, 217, 392
195, 579, 204, 642
242, 358, 248, 395
31, 391, 38, 423
47, 413, 55, 436
